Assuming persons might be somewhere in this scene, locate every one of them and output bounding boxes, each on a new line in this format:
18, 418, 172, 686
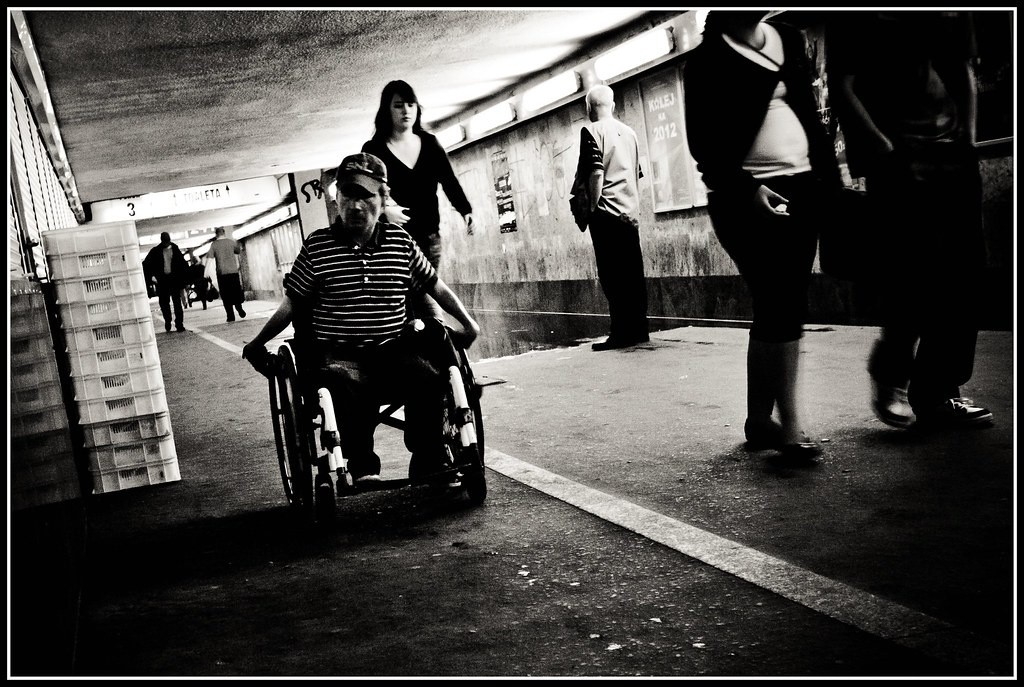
824, 11, 998, 437
680, 9, 847, 474
203, 227, 247, 323
361, 78, 476, 280
567, 82, 654, 352
147, 231, 194, 334
178, 255, 213, 311
240, 153, 482, 494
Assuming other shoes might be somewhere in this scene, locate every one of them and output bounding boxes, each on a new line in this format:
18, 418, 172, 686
870, 378, 913, 427
775, 439, 825, 459
744, 416, 784, 444
591, 338, 625, 350
912, 397, 994, 425
347, 452, 383, 481
237, 307, 246, 318
165, 316, 172, 331
177, 327, 185, 332
227, 317, 235, 321
409, 452, 451, 477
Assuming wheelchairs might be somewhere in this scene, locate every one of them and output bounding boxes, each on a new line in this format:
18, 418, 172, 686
268, 318, 490, 508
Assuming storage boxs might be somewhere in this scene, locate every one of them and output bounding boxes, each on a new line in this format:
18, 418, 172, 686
10, 220, 183, 504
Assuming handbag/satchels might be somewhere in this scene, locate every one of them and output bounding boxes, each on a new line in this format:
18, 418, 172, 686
210, 281, 218, 299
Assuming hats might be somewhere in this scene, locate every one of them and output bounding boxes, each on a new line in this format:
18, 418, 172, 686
335, 152, 388, 194
